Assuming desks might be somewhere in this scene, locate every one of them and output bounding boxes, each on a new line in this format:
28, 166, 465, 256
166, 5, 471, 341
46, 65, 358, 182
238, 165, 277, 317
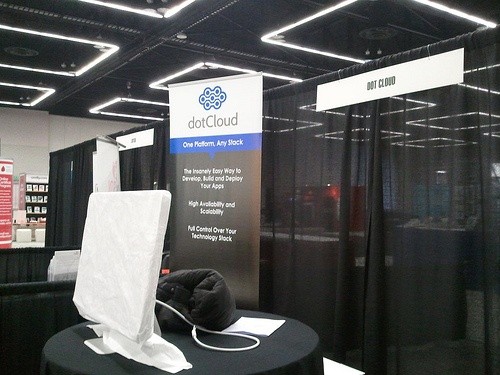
259, 223, 478, 249
41, 310, 325, 375
